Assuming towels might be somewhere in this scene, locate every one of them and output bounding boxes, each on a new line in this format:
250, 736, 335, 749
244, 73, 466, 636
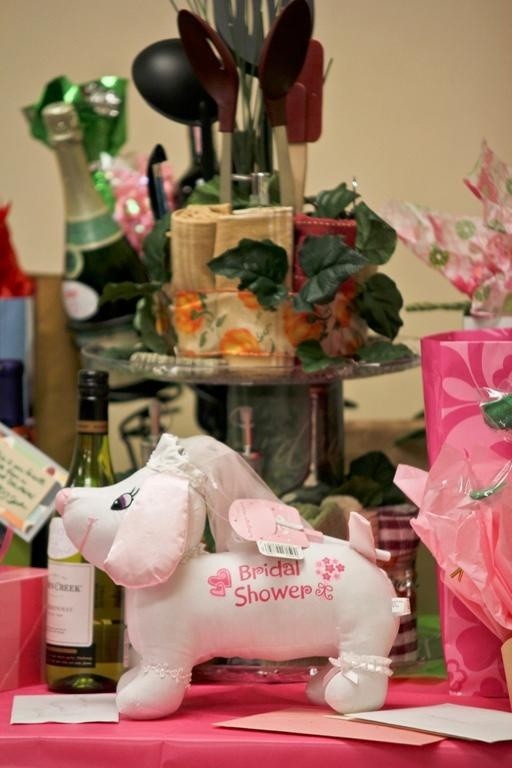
170, 202, 357, 371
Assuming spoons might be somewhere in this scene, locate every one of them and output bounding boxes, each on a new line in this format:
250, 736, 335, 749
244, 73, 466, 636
178, 0, 313, 212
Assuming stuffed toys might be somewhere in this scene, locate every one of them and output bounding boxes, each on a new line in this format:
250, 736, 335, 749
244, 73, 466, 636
52, 426, 415, 721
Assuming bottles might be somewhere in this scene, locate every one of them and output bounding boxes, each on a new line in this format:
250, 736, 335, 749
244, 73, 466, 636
0, 358, 38, 567
37, 99, 145, 333
42, 370, 124, 691
